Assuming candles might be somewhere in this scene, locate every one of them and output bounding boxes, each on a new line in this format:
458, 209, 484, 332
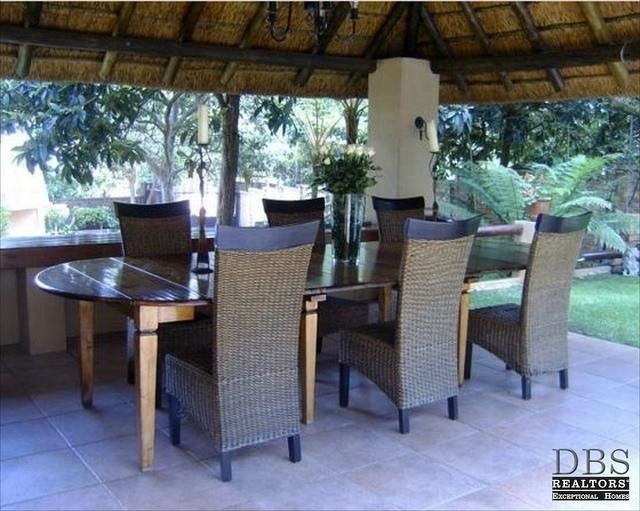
197, 102, 209, 146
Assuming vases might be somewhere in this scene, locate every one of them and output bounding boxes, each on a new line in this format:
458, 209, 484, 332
328, 193, 364, 265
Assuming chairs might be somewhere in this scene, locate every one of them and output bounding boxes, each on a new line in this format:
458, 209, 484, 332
113, 195, 593, 483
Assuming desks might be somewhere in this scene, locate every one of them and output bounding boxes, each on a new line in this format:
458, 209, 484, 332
31, 228, 624, 472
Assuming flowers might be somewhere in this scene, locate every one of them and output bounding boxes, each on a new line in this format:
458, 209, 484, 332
306, 134, 380, 233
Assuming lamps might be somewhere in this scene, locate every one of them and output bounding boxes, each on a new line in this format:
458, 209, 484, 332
415, 115, 441, 155
265, 0, 362, 45
